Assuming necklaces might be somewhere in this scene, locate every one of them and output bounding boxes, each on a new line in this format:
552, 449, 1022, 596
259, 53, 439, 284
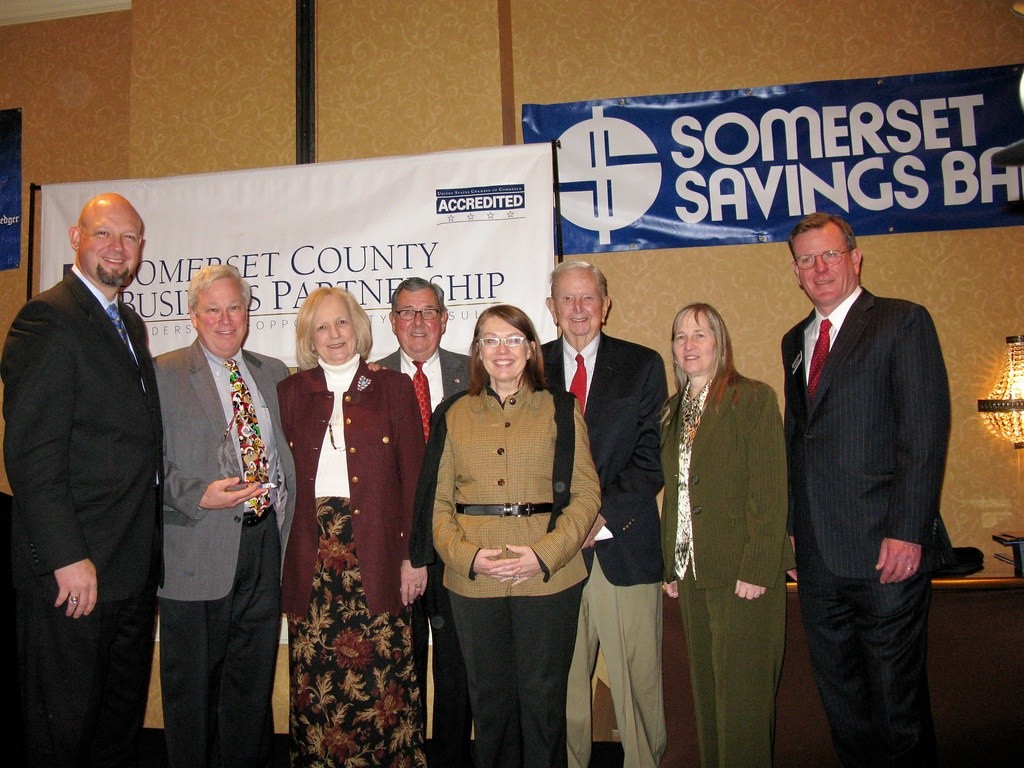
328, 423, 347, 452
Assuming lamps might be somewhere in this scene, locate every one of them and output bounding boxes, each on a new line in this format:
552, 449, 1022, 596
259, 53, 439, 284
979, 336, 1024, 576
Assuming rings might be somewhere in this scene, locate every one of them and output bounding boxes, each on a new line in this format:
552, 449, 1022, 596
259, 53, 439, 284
69, 596, 79, 604
415, 584, 422, 588
906, 566, 914, 570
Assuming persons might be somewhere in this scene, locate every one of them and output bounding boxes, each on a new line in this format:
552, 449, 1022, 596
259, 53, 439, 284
540, 260, 667, 768
780, 211, 951, 768
0, 193, 163, 768
152, 264, 297, 768
278, 286, 428, 768
409, 307, 599, 768
370, 278, 488, 768
657, 303, 795, 768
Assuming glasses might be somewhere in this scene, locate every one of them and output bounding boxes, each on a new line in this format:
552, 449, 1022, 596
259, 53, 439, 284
795, 249, 854, 270
479, 337, 530, 348
395, 309, 441, 321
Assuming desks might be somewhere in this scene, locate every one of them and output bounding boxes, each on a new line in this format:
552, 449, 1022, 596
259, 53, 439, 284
592, 577, 1024, 768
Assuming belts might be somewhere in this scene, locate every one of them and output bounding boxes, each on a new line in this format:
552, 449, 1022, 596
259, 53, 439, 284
242, 504, 273, 527
456, 501, 554, 518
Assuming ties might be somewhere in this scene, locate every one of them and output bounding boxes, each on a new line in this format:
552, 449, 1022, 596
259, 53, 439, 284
808, 319, 833, 404
412, 360, 432, 447
570, 353, 587, 418
221, 359, 271, 519
105, 302, 137, 369
